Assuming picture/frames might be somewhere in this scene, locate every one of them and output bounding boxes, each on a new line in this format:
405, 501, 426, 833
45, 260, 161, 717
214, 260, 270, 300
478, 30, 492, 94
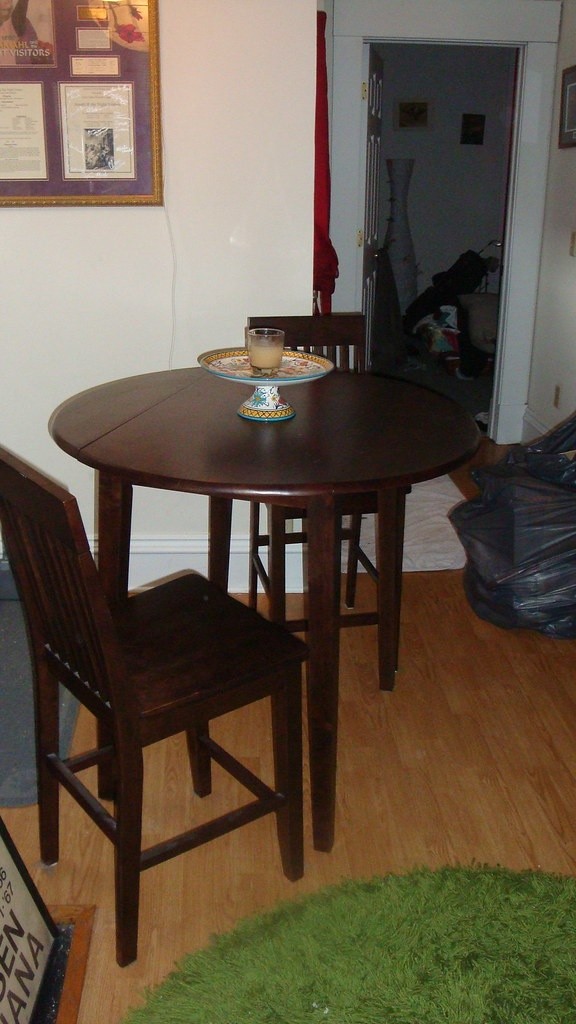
392, 98, 434, 131
1, 0, 163, 207
558, 65, 576, 147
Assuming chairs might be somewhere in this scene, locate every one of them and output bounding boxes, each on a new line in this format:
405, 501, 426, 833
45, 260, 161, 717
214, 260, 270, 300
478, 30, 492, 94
246, 313, 412, 673
0, 443, 312, 969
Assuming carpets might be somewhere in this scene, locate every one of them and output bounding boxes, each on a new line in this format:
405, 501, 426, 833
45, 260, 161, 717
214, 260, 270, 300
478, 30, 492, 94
124, 864, 576, 1024
0, 596, 79, 809
341, 474, 468, 573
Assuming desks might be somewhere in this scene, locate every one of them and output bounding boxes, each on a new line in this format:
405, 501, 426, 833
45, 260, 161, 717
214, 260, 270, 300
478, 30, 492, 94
49, 365, 482, 854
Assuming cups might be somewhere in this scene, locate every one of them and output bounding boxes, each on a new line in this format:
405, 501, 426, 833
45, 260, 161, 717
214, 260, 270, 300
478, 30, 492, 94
247, 328, 285, 375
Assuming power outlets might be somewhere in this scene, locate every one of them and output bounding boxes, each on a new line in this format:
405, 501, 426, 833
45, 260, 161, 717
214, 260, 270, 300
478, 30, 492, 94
553, 385, 561, 408
